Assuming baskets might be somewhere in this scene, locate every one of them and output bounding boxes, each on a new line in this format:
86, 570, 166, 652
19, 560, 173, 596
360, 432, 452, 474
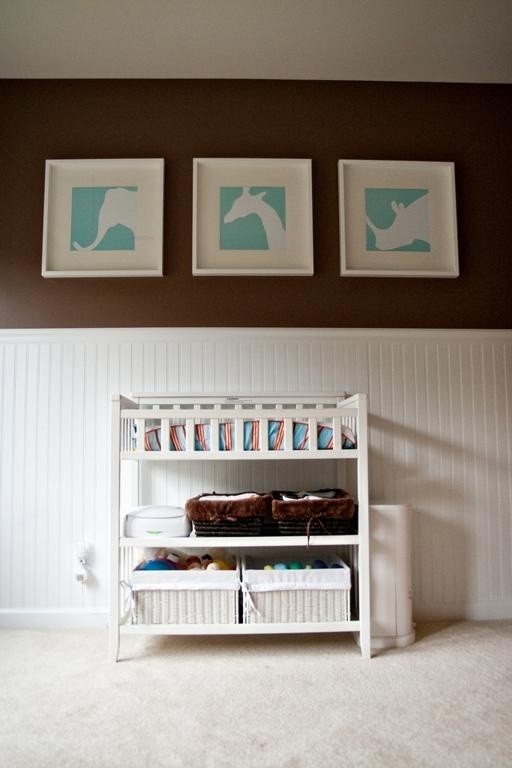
130, 551, 241, 625
240, 552, 352, 625
270, 489, 355, 536
187, 491, 270, 537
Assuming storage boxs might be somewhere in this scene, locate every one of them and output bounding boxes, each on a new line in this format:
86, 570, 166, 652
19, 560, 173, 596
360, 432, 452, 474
122, 546, 352, 632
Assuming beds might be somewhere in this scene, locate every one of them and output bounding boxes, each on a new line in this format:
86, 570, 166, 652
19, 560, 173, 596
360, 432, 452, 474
107, 388, 374, 664
